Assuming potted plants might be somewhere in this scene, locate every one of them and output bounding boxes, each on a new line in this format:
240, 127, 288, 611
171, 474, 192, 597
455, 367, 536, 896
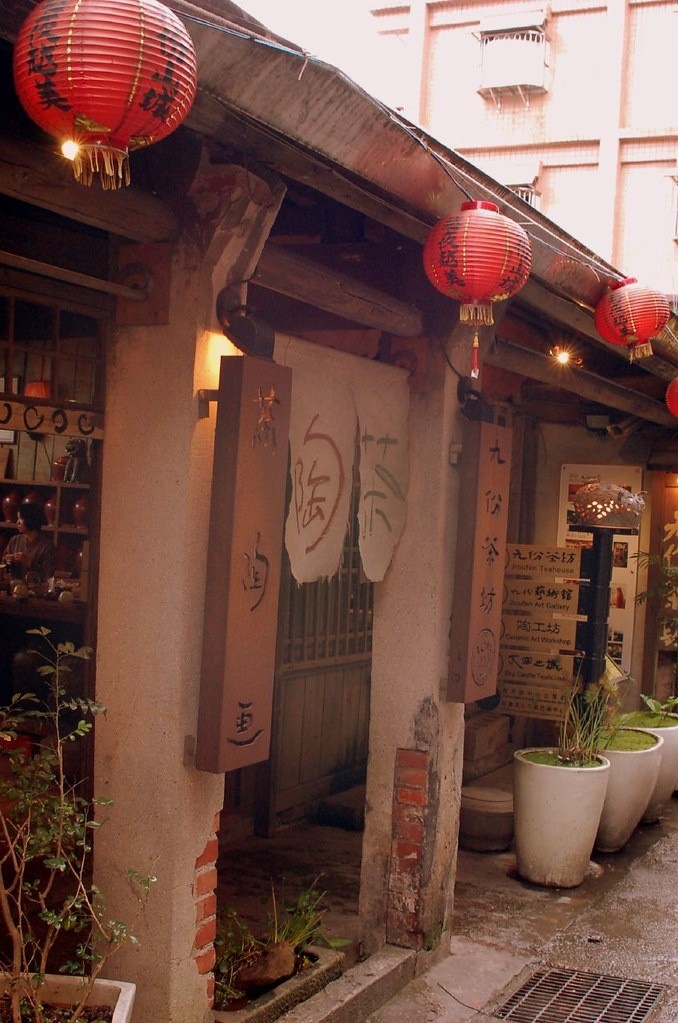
512, 653, 677, 889
0, 628, 160, 1022
214, 864, 350, 1021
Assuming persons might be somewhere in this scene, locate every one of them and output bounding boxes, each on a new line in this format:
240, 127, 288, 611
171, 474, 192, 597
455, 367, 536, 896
3, 503, 73, 582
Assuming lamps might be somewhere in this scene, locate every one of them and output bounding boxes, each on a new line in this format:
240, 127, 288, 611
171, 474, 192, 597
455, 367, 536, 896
217, 273, 274, 363
458, 378, 495, 423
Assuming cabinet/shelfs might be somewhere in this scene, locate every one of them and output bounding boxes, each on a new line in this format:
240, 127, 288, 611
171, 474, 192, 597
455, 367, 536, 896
0, 479, 90, 581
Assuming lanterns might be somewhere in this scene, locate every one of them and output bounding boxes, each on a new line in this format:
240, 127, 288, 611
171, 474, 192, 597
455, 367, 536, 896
592, 278, 670, 361
13, 0, 198, 191
422, 198, 531, 374
666, 378, 678, 418
